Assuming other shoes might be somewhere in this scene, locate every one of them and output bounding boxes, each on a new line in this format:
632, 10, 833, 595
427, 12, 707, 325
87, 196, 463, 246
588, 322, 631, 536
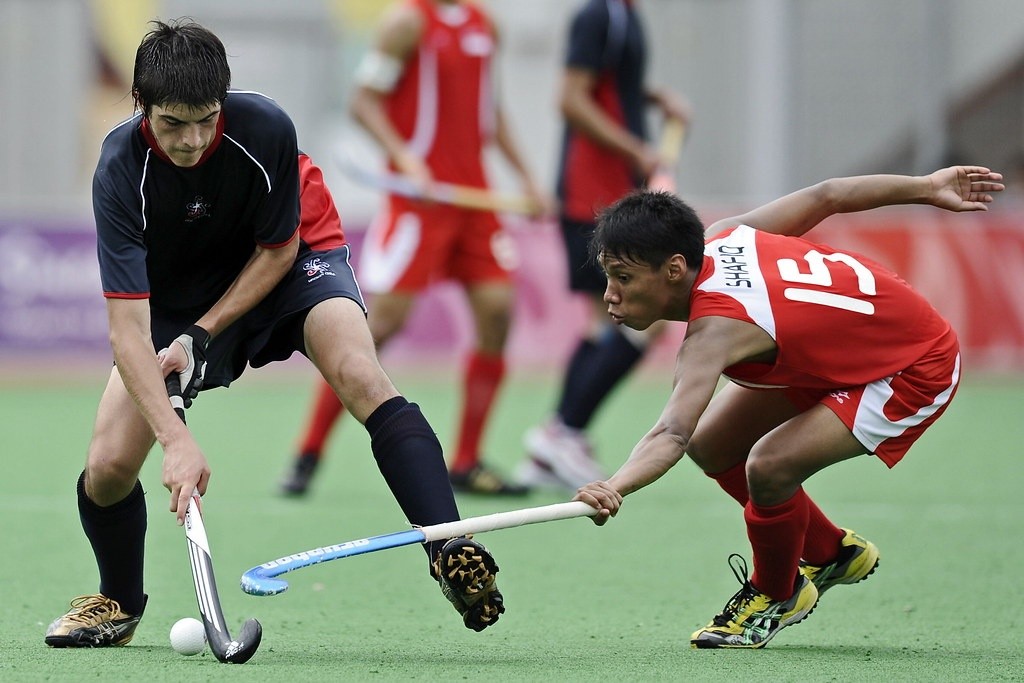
523, 414, 608, 494
448, 458, 534, 496
279, 450, 321, 495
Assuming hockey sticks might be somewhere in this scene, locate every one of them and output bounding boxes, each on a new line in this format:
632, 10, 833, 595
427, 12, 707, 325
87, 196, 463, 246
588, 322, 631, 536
155, 347, 264, 667
237, 499, 601, 599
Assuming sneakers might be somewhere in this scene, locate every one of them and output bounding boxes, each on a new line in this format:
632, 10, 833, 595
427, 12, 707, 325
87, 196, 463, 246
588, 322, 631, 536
796, 522, 884, 600
433, 530, 507, 633
691, 552, 823, 649
44, 592, 149, 649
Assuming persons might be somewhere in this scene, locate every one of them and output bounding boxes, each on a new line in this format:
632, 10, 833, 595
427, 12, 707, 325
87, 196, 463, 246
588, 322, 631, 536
44, 20, 506, 649
573, 166, 1005, 649
525, 0, 689, 496
275, 0, 548, 501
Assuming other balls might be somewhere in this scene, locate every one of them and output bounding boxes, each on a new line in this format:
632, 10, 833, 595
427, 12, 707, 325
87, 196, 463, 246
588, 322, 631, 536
169, 617, 209, 657
